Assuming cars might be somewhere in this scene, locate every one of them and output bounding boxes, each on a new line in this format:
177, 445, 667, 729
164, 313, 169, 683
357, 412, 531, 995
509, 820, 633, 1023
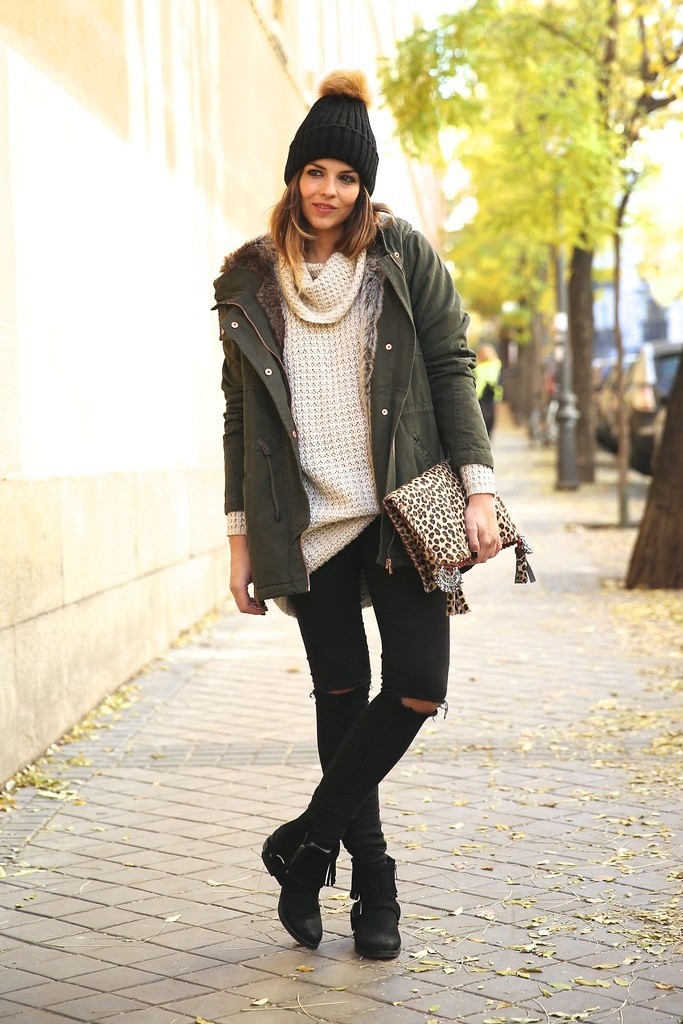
593, 341, 683, 475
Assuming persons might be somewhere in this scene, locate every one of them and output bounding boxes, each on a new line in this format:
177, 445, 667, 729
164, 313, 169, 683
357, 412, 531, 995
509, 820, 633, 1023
471, 345, 502, 442
211, 73, 503, 959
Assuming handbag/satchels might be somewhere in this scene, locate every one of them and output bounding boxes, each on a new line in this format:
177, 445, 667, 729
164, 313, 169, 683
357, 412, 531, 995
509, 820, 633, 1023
381, 457, 536, 616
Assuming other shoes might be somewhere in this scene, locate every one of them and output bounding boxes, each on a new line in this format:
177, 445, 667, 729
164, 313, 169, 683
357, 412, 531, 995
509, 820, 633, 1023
349, 853, 402, 958
261, 810, 340, 950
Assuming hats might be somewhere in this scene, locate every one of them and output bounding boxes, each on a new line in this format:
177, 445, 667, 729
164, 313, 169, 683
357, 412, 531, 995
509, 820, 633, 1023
284, 69, 381, 196
